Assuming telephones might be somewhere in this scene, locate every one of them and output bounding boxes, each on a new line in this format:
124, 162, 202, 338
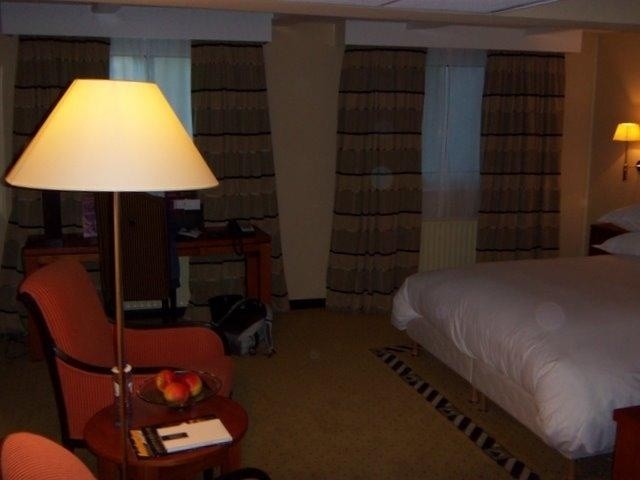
230, 218, 255, 235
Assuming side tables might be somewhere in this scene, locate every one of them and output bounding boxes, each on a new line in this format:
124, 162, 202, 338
83, 393, 249, 480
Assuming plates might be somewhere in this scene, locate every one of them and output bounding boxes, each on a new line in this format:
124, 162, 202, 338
136, 369, 223, 408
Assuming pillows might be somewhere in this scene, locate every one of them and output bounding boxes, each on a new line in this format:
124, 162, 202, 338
590, 204, 640, 258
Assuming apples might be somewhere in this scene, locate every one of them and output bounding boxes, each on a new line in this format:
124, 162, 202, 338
163, 383, 190, 403
181, 371, 202, 398
156, 370, 176, 393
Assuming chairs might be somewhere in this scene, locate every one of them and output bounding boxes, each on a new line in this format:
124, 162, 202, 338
16, 255, 232, 451
93, 192, 180, 322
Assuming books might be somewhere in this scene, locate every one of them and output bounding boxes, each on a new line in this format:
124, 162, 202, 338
128, 417, 234, 460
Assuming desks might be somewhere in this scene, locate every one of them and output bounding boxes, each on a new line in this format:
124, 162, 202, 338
21, 224, 275, 363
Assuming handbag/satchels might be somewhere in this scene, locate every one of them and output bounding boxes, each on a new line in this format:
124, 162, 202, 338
208, 284, 274, 358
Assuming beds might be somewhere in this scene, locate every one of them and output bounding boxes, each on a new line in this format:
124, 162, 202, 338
392, 254, 640, 478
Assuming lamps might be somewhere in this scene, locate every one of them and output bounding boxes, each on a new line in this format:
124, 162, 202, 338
6, 77, 223, 479
610, 122, 639, 182
